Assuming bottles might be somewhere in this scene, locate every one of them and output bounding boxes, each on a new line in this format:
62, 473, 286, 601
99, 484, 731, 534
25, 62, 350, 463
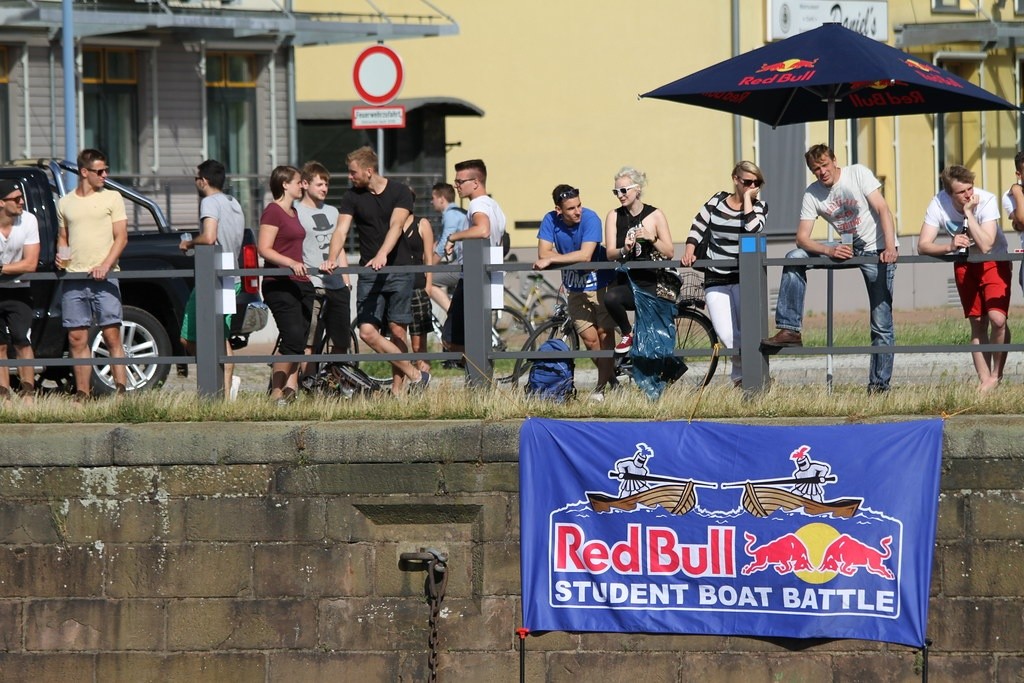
635, 221, 646, 261
959, 217, 970, 257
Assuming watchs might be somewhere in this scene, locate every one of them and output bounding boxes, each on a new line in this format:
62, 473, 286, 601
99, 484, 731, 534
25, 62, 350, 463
650, 236, 657, 244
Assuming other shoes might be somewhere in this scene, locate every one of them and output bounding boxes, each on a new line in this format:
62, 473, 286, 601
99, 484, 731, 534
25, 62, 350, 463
614, 334, 634, 353
116, 384, 127, 399
440, 361, 464, 370
75, 390, 90, 404
18, 382, 35, 402
0, 386, 11, 401
230, 376, 241, 402
409, 370, 432, 403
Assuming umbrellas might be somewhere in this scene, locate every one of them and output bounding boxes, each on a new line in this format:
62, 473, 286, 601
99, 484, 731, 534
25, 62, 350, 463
636, 22, 1022, 398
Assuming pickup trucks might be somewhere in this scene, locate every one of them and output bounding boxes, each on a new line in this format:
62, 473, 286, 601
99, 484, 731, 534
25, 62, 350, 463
0, 159, 272, 395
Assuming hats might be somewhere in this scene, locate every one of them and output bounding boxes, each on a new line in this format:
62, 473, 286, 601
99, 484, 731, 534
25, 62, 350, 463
0, 180, 21, 199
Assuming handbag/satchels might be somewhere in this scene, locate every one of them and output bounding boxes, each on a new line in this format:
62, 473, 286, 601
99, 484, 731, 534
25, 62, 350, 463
691, 192, 729, 271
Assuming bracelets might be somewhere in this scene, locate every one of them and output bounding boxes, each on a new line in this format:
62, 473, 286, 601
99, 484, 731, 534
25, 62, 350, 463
620, 246, 626, 257
446, 233, 456, 243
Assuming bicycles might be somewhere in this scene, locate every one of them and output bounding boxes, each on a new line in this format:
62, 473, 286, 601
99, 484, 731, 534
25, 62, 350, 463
512, 279, 719, 393
352, 286, 536, 386
266, 320, 359, 396
491, 256, 571, 341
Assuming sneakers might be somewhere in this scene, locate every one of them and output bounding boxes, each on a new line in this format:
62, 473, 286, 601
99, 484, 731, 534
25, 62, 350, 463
761, 329, 804, 347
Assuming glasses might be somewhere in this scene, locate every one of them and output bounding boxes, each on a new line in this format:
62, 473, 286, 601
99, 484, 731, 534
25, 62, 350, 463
560, 188, 580, 199
611, 185, 637, 195
454, 179, 475, 186
194, 177, 203, 182
738, 177, 762, 187
87, 168, 111, 176
3, 194, 25, 203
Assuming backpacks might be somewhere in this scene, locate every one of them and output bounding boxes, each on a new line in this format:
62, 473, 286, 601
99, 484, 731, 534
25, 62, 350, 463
523, 338, 577, 405
450, 207, 510, 257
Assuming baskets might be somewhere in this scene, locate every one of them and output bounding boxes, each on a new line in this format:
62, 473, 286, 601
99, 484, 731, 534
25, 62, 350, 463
676, 272, 708, 310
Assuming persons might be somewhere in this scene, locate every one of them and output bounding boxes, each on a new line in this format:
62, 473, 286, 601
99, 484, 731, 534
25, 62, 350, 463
918, 164, 1013, 395
762, 142, 899, 392
179, 158, 244, 405
532, 185, 621, 407
1002, 150, 1024, 291
443, 159, 505, 352
681, 160, 768, 389
296, 161, 355, 401
605, 168, 675, 354
0, 178, 40, 404
424, 182, 469, 311
318, 147, 431, 399
257, 165, 315, 405
402, 186, 434, 373
55, 149, 128, 403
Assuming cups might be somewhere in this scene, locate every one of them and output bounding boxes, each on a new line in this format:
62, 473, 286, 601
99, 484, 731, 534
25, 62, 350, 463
841, 233, 853, 252
180, 232, 195, 256
59, 246, 71, 268
